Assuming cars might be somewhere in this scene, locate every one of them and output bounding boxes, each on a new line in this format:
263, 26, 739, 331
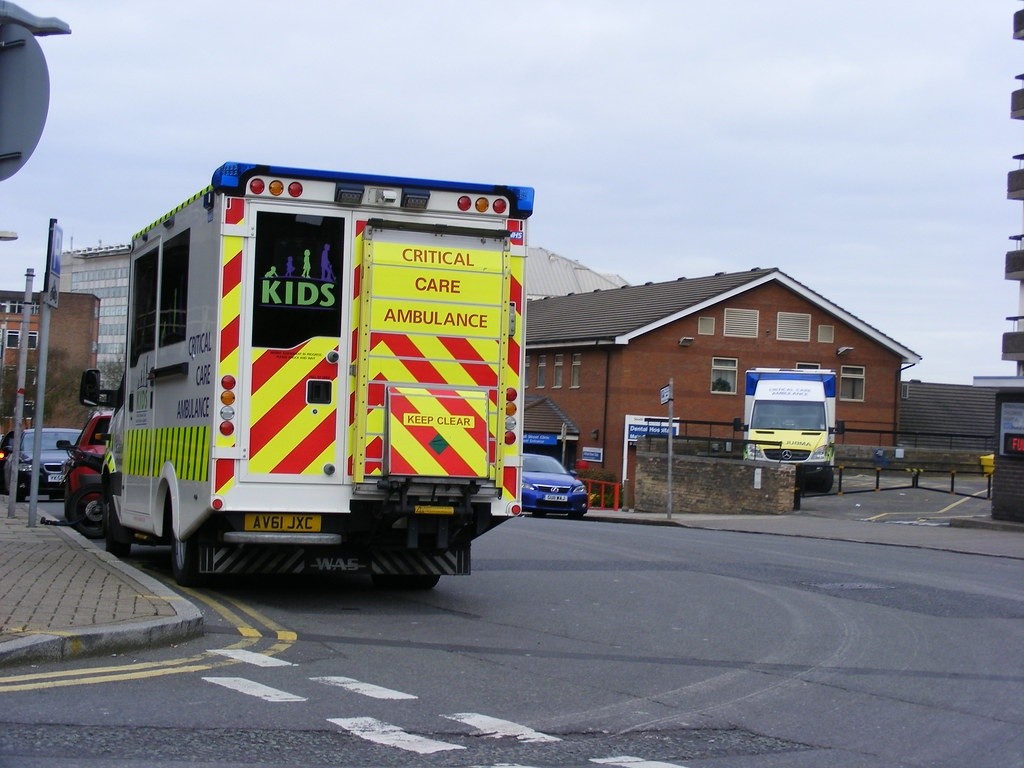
521, 452, 589, 519
2, 428, 83, 503
0, 431, 23, 477
56, 411, 119, 539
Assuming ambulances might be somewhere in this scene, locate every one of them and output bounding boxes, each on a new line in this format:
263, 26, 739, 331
732, 369, 846, 494
78, 160, 535, 584
